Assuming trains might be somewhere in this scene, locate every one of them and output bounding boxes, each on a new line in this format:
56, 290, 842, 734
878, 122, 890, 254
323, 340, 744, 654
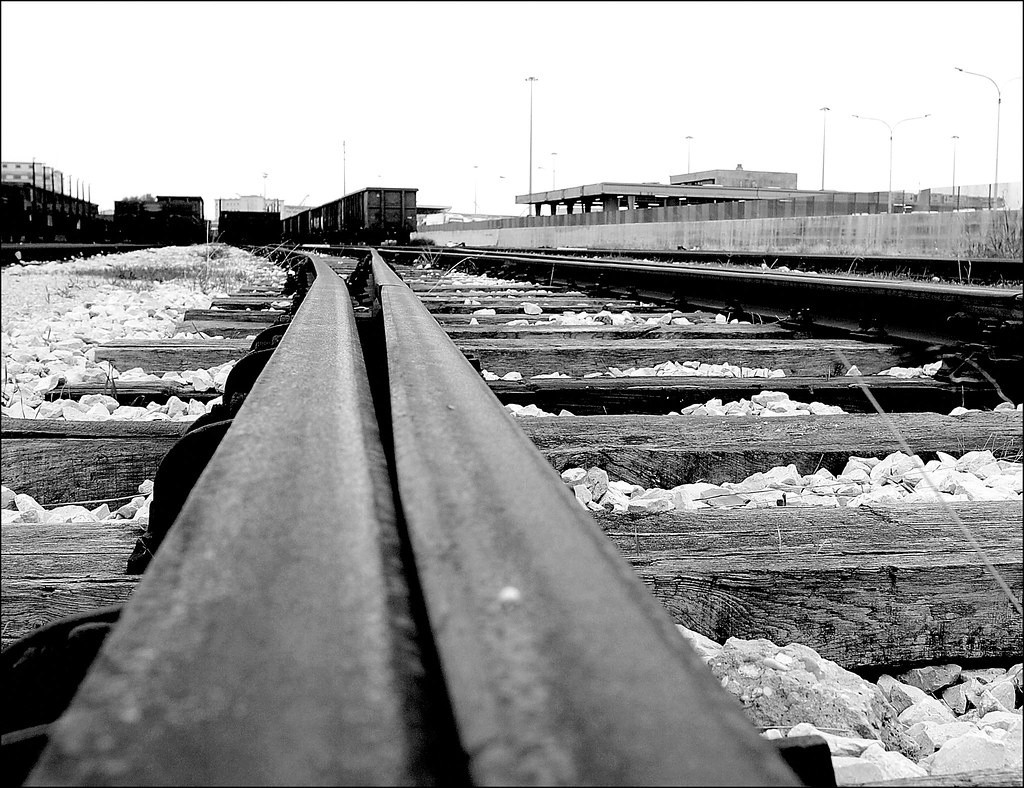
278, 187, 420, 242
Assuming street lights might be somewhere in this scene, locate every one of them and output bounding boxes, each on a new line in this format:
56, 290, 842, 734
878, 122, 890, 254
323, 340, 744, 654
524, 76, 539, 216
817, 107, 830, 191
954, 66, 1002, 209
849, 112, 933, 215
